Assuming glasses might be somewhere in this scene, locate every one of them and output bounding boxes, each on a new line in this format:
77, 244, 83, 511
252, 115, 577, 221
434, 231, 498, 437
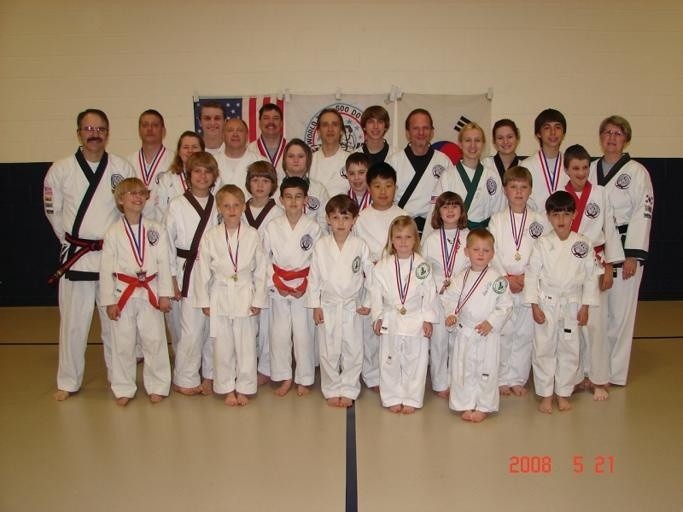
126, 190, 149, 196
78, 125, 107, 132
602, 130, 624, 137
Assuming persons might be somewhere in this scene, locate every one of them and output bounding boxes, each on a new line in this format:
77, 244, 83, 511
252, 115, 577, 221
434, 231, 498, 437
43, 101, 656, 423
